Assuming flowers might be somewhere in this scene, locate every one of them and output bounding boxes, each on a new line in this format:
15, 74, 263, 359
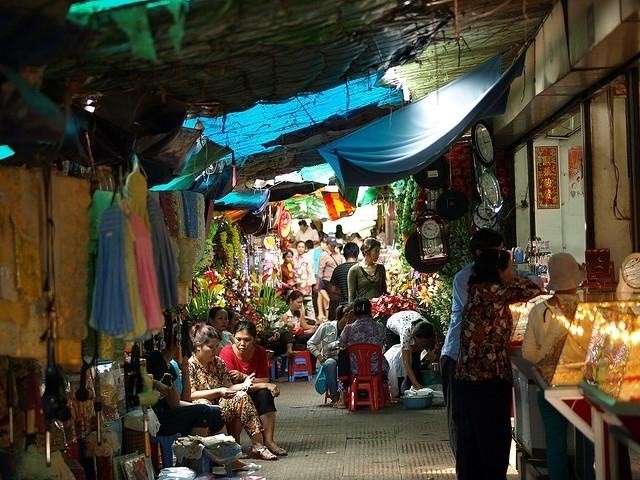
186, 269, 226, 324
256, 283, 294, 342
409, 270, 444, 342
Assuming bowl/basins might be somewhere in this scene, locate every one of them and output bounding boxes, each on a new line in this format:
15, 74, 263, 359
400, 396, 433, 409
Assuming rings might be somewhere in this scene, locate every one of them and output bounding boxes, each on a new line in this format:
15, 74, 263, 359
321, 357, 323, 360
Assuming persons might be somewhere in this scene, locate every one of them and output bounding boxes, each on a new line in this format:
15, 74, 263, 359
334, 243, 347, 265
331, 242, 360, 304
294, 220, 319, 244
332, 225, 347, 244
449, 248, 541, 480
182, 323, 277, 461
331, 297, 385, 409
295, 241, 317, 325
349, 232, 364, 261
314, 237, 339, 320
306, 304, 355, 407
346, 238, 391, 305
139, 349, 246, 471
281, 249, 297, 297
208, 307, 236, 356
224, 303, 246, 336
279, 291, 317, 357
385, 309, 437, 404
218, 320, 287, 456
162, 339, 224, 410
440, 228, 504, 456
522, 253, 595, 480
305, 239, 324, 316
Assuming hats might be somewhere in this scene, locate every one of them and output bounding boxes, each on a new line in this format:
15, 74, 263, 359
545, 253, 588, 290
326, 236, 338, 246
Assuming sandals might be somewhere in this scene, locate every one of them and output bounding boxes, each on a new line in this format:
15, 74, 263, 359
274, 447, 287, 456
250, 446, 278, 460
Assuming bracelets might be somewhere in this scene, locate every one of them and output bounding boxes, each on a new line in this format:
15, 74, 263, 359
415, 384, 421, 388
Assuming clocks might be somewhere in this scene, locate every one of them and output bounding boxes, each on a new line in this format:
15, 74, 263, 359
277, 211, 291, 237
477, 202, 497, 219
471, 120, 495, 167
473, 212, 498, 229
478, 170, 502, 210
415, 208, 449, 265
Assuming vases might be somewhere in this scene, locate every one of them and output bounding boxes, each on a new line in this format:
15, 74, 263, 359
188, 325, 195, 352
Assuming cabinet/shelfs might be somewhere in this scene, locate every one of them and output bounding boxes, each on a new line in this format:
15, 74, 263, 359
580, 305, 640, 417
509, 293, 572, 458
531, 292, 640, 480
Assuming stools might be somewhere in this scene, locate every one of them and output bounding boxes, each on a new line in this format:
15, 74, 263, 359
348, 383, 373, 410
152, 433, 181, 469
268, 360, 276, 381
183, 454, 233, 478
288, 350, 312, 383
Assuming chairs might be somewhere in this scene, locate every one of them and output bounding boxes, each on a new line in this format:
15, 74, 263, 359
320, 348, 331, 405
346, 343, 383, 411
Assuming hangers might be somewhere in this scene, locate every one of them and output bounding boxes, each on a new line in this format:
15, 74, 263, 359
109, 175, 124, 208
